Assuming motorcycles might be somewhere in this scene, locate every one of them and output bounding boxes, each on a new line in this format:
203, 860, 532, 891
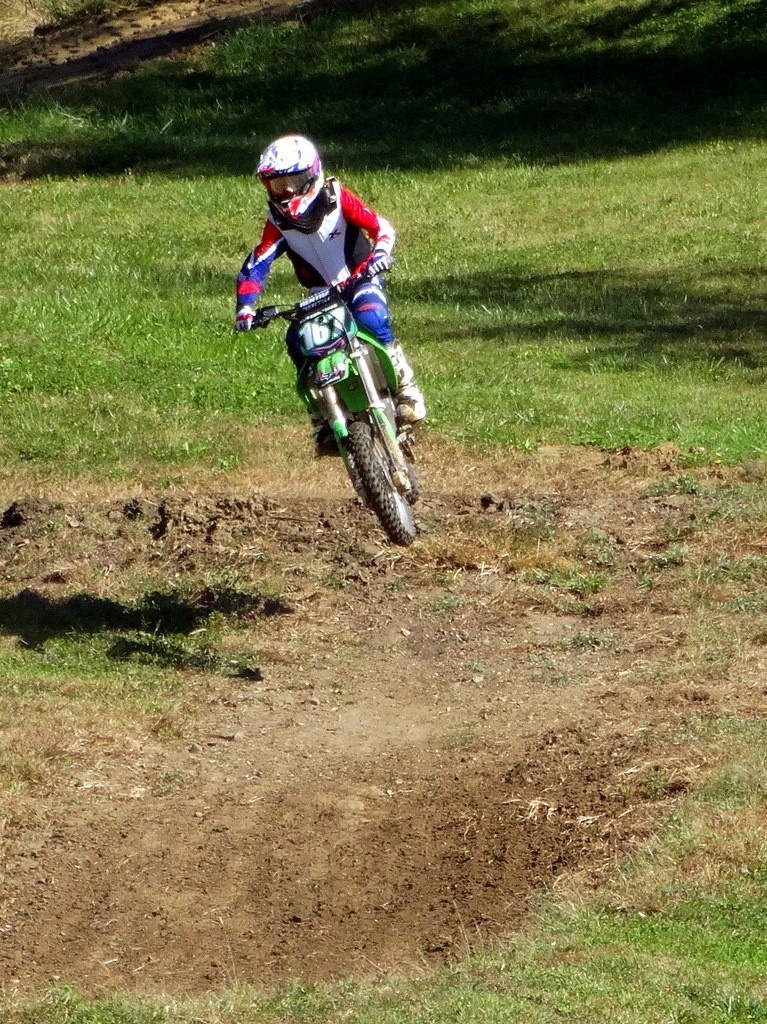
250, 256, 424, 548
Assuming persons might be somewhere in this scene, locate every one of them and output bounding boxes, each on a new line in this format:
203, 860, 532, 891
233, 135, 428, 458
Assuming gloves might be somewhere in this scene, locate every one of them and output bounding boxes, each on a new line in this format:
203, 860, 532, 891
234, 305, 257, 330
362, 252, 391, 276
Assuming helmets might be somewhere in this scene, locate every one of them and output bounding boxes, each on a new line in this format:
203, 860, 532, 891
258, 134, 323, 223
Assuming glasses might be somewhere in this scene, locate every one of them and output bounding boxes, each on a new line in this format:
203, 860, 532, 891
268, 169, 313, 198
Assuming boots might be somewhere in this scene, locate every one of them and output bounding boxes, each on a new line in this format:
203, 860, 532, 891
307, 403, 339, 458
385, 336, 426, 422
